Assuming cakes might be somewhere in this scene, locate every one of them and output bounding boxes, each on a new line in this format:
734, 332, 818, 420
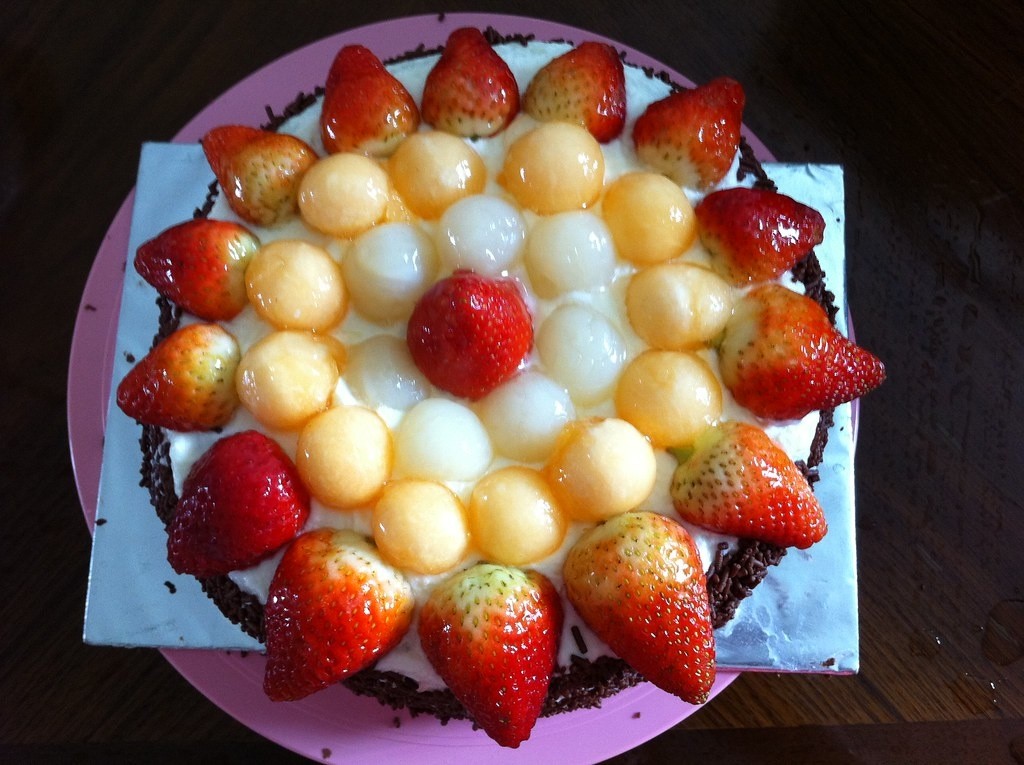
115, 22, 887, 752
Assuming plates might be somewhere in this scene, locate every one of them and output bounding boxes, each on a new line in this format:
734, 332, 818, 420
66, 12, 861, 765
